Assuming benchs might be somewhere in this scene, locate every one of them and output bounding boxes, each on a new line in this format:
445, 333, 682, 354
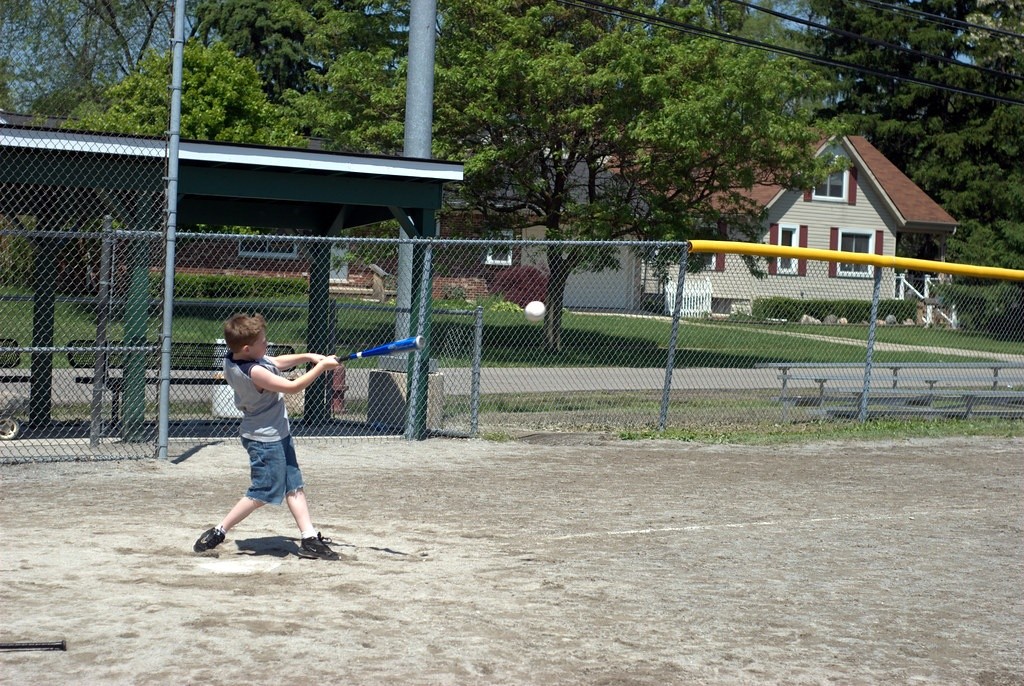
67, 340, 297, 431
0, 338, 35, 384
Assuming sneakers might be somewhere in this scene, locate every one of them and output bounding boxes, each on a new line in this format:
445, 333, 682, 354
192, 526, 225, 553
298, 532, 342, 560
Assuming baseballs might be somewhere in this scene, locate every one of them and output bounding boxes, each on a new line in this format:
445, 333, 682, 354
524, 301, 546, 321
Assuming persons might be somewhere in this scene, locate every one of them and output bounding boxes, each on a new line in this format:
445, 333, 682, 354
192, 313, 343, 560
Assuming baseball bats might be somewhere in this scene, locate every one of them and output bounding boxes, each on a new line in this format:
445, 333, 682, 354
335, 335, 426, 363
